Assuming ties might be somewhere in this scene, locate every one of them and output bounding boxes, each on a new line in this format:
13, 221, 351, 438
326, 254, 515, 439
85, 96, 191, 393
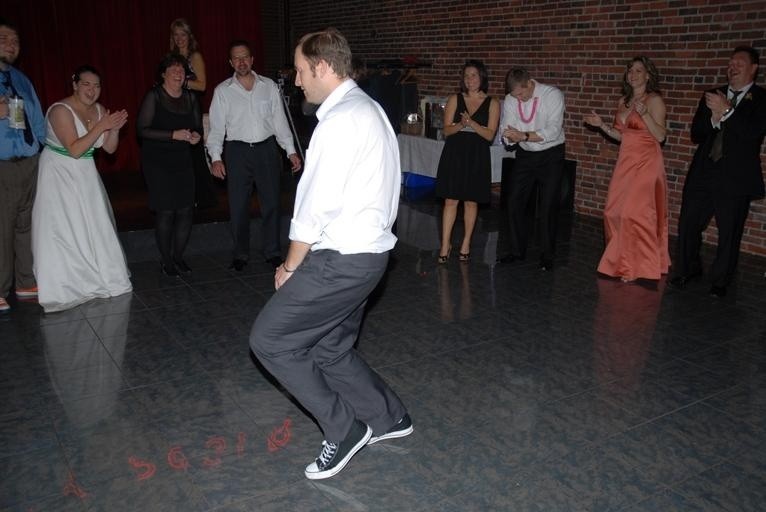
2, 71, 34, 146
709, 89, 743, 162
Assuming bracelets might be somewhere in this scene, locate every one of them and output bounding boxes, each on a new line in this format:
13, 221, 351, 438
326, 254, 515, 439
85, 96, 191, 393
184, 79, 190, 91
722, 105, 733, 116
607, 124, 612, 136
523, 131, 529, 142
281, 263, 296, 273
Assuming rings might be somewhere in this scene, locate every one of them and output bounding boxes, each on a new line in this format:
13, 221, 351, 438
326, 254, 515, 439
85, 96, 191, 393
186, 135, 189, 139
466, 115, 470, 122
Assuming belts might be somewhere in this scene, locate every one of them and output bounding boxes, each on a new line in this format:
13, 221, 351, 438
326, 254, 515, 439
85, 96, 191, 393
234, 140, 268, 147
11, 156, 27, 161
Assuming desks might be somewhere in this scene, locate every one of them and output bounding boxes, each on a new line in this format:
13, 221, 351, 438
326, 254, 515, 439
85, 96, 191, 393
396, 133, 516, 199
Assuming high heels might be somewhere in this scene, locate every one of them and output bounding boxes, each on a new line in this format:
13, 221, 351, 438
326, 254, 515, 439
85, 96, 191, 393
459, 245, 471, 261
438, 244, 452, 263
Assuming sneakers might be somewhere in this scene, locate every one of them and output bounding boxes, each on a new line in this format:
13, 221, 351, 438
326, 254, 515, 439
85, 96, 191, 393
305, 418, 373, 480
0, 297, 10, 310
367, 413, 413, 445
16, 288, 37, 296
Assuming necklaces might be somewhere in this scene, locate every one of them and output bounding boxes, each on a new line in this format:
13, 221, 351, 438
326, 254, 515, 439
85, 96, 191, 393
77, 99, 97, 126
517, 97, 539, 123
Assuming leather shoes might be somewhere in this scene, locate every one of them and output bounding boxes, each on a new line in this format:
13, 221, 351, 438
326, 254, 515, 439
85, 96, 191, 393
162, 262, 192, 279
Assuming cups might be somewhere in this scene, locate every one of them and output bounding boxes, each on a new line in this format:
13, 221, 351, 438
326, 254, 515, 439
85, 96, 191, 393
1, 93, 25, 130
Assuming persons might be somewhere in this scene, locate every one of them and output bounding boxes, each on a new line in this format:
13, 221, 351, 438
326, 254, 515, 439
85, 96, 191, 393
667, 45, 765, 301
206, 42, 301, 273
590, 271, 670, 397
500, 67, 567, 272
134, 56, 204, 276
0, 22, 44, 308
155, 20, 218, 209
433, 58, 501, 265
250, 24, 414, 482
434, 262, 475, 349
37, 286, 137, 498
583, 56, 672, 285
29, 66, 132, 313
